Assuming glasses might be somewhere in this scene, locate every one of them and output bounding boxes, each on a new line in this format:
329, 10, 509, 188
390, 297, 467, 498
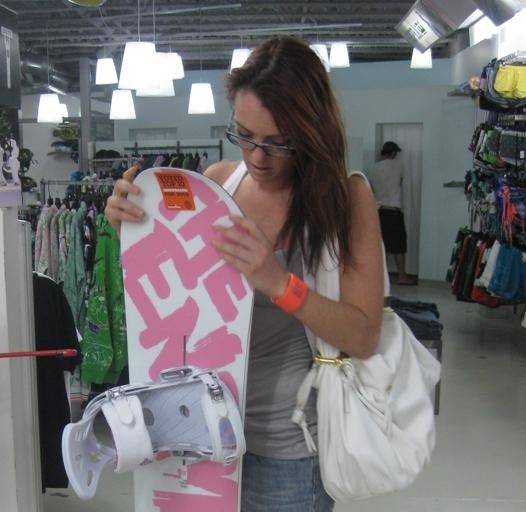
226, 110, 295, 159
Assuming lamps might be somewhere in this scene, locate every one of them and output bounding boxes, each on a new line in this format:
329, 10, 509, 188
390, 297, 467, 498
118, 1, 154, 91
37, 25, 64, 123
59, 102, 69, 118
230, 34, 252, 75
187, 3, 217, 116
136, 1, 176, 98
94, 8, 119, 86
411, 47, 433, 71
109, 88, 136, 121
329, 8, 350, 70
309, 29, 330, 72
164, 34, 185, 80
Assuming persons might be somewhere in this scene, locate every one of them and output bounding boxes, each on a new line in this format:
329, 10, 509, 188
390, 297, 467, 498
368, 141, 416, 285
103, 36, 387, 512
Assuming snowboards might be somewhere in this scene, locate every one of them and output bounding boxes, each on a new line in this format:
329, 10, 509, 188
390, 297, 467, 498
61, 167, 254, 512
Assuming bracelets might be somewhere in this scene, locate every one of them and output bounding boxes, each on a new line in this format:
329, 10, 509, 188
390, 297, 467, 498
269, 273, 307, 312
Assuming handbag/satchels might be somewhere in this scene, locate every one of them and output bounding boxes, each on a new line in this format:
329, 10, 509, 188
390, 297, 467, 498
291, 307, 441, 501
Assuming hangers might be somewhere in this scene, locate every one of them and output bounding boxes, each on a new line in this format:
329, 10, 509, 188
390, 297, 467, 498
96, 148, 207, 179
41, 181, 112, 212
